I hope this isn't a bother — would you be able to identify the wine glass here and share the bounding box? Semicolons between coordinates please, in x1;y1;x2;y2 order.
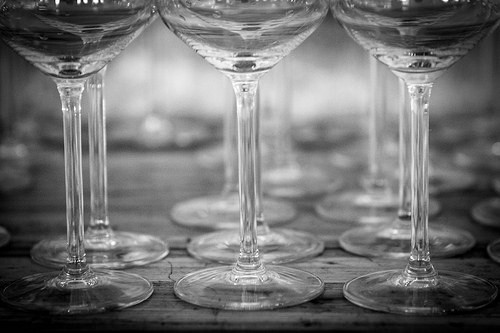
0;0;500;316
